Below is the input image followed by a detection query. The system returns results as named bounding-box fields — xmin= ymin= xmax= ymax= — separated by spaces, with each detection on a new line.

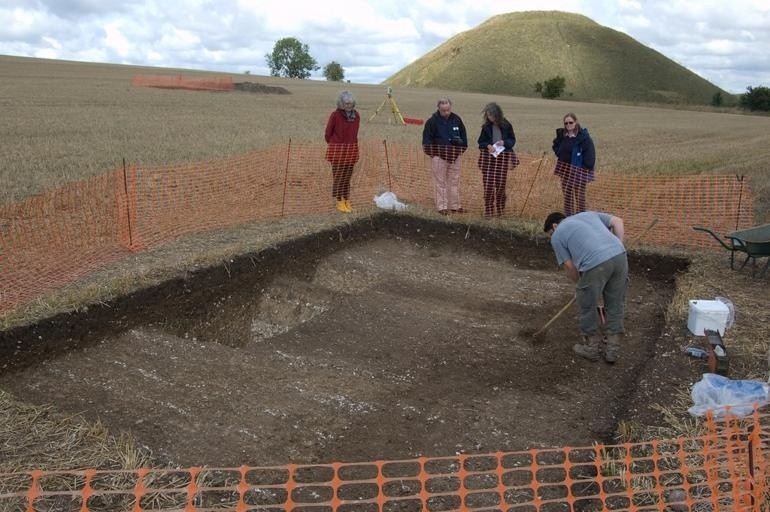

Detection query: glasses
xmin=564 ymin=122 xmax=574 ymax=125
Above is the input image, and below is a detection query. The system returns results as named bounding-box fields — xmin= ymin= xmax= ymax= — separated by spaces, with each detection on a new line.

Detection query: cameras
xmin=449 ymin=137 xmax=466 ymax=148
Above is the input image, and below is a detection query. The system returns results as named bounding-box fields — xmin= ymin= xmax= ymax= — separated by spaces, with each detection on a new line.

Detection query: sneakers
xmin=336 ymin=200 xmax=353 ymax=214
xmin=451 ymin=209 xmax=467 ymax=214
xmin=439 ymin=209 xmax=448 ymax=215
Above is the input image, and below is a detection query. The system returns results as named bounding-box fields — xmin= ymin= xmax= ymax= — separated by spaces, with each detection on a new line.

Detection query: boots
xmin=601 ymin=334 xmax=625 ymax=362
xmin=574 ymin=335 xmax=599 ymax=362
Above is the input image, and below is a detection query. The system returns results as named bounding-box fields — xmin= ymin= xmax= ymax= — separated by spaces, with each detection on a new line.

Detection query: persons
xmin=543 ymin=211 xmax=629 ymax=362
xmin=422 ymin=98 xmax=468 ymax=216
xmin=477 ymin=101 xmax=520 ymax=220
xmin=325 ymin=91 xmax=360 ymax=213
xmin=553 ymin=113 xmax=596 ymax=215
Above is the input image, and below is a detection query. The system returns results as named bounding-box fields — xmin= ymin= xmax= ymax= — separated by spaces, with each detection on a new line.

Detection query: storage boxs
xmin=687 ymin=299 xmax=729 ymax=337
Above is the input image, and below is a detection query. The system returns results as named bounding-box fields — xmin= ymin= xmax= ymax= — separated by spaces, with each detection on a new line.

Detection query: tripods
xmin=368 ymin=96 xmax=406 ymax=125
